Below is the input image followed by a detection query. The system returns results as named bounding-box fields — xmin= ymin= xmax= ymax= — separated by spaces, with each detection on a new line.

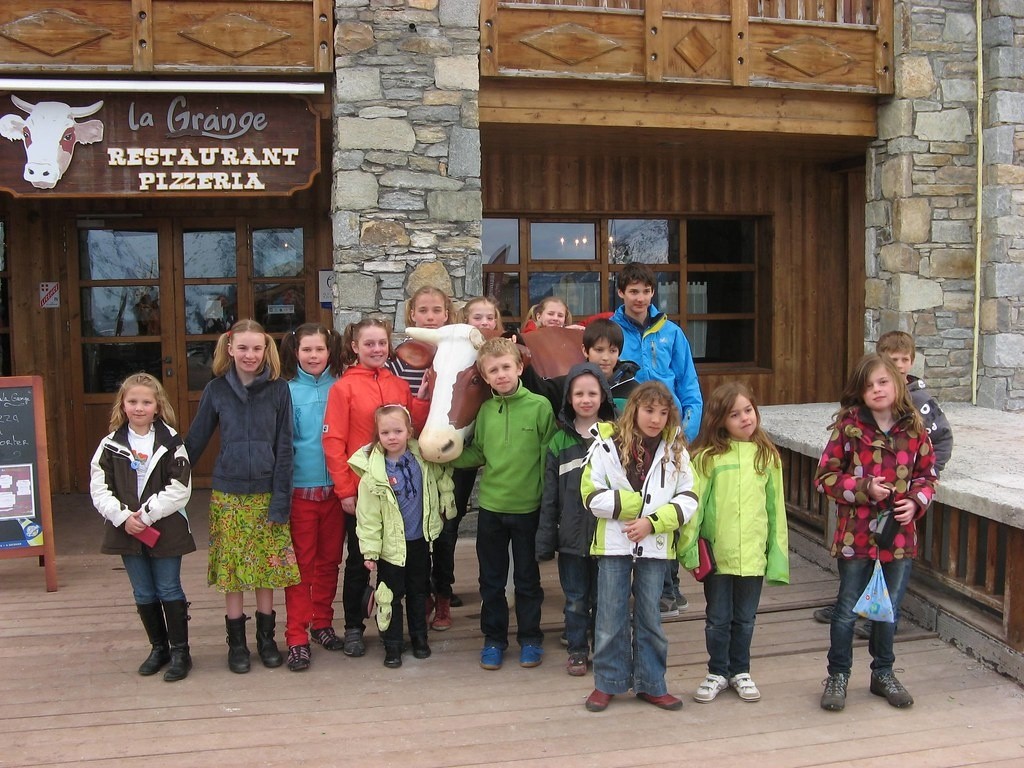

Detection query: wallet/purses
xmin=874 ymin=492 xmax=901 ymax=549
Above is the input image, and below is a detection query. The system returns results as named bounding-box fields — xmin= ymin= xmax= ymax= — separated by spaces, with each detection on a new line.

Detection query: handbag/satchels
xmin=853 ymin=559 xmax=894 ymax=624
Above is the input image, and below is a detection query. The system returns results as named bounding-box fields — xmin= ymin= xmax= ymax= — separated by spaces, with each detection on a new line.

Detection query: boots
xmin=137 ymin=607 xmax=170 ymax=675
xmin=226 ymin=613 xmax=250 ymax=673
xmin=255 ymin=610 xmax=282 ymax=667
xmin=162 ymin=600 xmax=192 ymax=681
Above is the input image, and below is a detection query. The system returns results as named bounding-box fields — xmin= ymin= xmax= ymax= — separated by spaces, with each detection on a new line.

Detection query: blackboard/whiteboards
xmin=0 ymin=376 xmax=53 ymax=559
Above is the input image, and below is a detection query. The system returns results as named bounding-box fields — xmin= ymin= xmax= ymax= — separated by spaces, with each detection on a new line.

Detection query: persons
xmin=813 ymin=353 xmax=936 ymax=713
xmin=181 ymin=262 xmax=702 ymax=676
xmin=814 ymin=332 xmax=953 ymax=639
xmin=356 ymin=404 xmax=455 ymax=668
xmin=676 ymin=382 xmax=788 ymax=704
xmin=89 ymin=373 xmax=192 ymax=681
xmin=580 ymin=383 xmax=700 ymax=712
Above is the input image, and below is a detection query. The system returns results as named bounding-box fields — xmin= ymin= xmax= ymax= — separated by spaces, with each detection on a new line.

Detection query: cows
xmin=394 ymin=321 xmax=586 ymax=462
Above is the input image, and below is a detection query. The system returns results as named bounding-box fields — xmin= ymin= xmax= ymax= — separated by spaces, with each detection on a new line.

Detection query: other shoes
xmin=814 ymin=606 xmax=835 ymax=622
xmin=855 ymin=621 xmax=872 ymax=638
xmin=449 ymin=592 xmax=463 ymax=606
xmin=676 ymin=593 xmax=690 ymax=609
xmin=659 ymin=596 xmax=679 ymax=618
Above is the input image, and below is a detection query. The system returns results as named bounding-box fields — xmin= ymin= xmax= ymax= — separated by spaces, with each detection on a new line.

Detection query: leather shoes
xmin=385 ymin=646 xmax=401 ymax=667
xmin=411 ymin=634 xmax=431 ymax=658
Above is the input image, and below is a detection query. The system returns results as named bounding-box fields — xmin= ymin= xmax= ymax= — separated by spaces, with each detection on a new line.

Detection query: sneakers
xmin=729 ymin=673 xmax=760 ymax=702
xmin=567 ymin=652 xmax=587 ymax=675
xmin=586 ymin=689 xmax=613 ymax=712
xmin=870 ymin=668 xmax=914 ymax=707
xmin=694 ymin=673 xmax=728 ymax=703
xmin=310 ymin=628 xmax=344 ymax=650
xmin=821 ymin=673 xmax=848 ymax=710
xmin=287 ymin=644 xmax=311 ymax=670
xmin=480 ymin=644 xmax=503 ymax=669
xmin=344 ymin=628 xmax=366 ymax=656
xmin=433 ymin=593 xmax=451 ymax=630
xmin=520 ymin=642 xmax=543 ymax=666
xmin=425 ymin=596 xmax=434 ymax=630
xmin=637 ymin=693 xmax=683 ymax=710
